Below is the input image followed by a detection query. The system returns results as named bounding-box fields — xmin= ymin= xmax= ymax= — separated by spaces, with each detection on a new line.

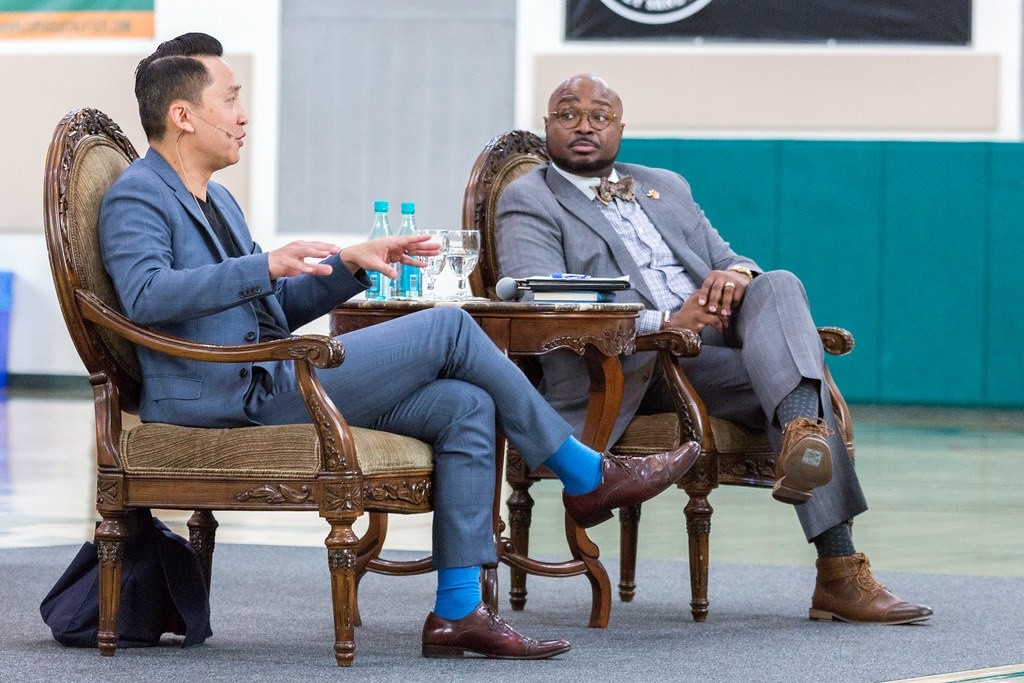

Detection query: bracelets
xmin=662 ymin=310 xmax=671 ymax=328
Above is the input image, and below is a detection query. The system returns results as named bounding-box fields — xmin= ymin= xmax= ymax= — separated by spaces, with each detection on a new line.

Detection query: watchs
xmin=727 ymin=266 xmax=753 ymax=281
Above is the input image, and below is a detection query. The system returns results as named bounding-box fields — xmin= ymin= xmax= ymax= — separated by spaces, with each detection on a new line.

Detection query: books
xmin=516 ymin=274 xmax=634 ymax=303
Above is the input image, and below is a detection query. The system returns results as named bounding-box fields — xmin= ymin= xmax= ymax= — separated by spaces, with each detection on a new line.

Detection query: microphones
xmin=495 ymin=277 xmax=525 ymax=302
xmin=187 ymin=106 xmax=234 ymax=137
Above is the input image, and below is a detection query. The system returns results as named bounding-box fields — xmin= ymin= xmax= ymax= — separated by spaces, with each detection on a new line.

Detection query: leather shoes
xmin=808 ymin=552 xmax=935 ymax=625
xmin=562 ymin=441 xmax=702 ymax=529
xmin=771 ymin=417 xmax=833 ymax=507
xmin=421 ymin=600 xmax=572 ymax=659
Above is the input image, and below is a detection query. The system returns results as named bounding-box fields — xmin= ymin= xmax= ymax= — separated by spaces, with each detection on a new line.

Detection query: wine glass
xmin=416 ymin=230 xmax=450 ymax=299
xmin=449 ymin=230 xmax=480 ymax=297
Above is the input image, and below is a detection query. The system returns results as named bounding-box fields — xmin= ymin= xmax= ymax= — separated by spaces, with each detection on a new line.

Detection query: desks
xmin=330 ymin=296 xmax=644 ymax=627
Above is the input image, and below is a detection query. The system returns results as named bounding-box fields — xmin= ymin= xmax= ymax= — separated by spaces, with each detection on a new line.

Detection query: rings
xmin=725 ymin=282 xmax=736 ymax=288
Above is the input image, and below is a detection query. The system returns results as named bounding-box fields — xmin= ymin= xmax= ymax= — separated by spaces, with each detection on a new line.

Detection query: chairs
xmin=463 ymin=129 xmax=854 ymax=622
xmin=43 ymin=106 xmax=435 ymax=666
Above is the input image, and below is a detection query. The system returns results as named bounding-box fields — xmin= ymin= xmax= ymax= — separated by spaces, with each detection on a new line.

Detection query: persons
xmin=97 ymin=33 xmax=700 ymax=659
xmin=495 ymin=76 xmax=935 ymax=628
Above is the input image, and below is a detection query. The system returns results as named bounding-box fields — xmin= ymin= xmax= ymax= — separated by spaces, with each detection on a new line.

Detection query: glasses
xmin=548 ymin=107 xmax=621 ymax=131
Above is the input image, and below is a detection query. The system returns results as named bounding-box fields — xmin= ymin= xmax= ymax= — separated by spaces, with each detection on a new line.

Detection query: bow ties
xmin=589 ymin=173 xmax=635 ymax=205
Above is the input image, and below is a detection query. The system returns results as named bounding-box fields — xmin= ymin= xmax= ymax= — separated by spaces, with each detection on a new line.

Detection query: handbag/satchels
xmin=43 ymin=509 xmax=214 ymax=651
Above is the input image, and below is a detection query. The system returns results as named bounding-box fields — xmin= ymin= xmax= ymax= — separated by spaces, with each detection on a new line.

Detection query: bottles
xmin=396 ymin=202 xmax=422 ymax=298
xmin=365 ymin=200 xmax=396 ymax=300
